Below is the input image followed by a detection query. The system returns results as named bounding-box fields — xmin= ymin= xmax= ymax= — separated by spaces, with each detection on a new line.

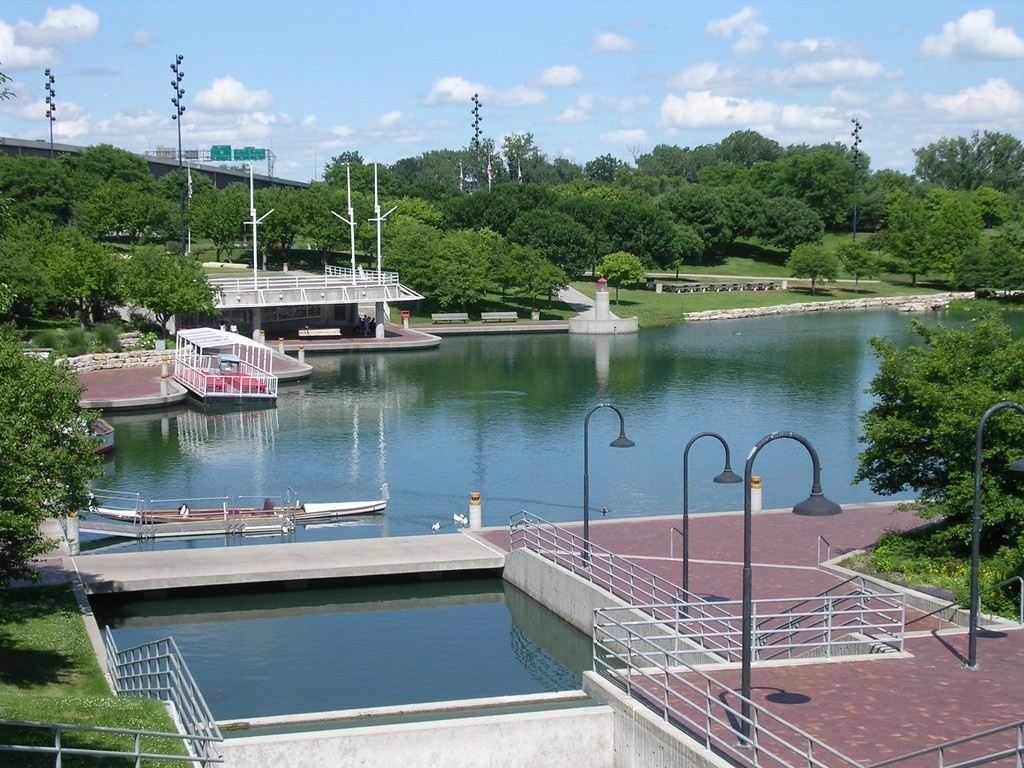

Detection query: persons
xmin=354 ymin=315 xmax=375 ymax=336
xmin=230 ymin=321 xmax=238 ymax=333
xmin=219 ymin=320 xmax=228 ymax=331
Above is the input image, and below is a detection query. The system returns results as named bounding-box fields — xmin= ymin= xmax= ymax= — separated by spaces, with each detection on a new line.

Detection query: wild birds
xmin=733 ymin=331 xmax=742 ymax=336
xmin=432 ymin=522 xmax=440 ymax=531
xmin=454 ymin=513 xmax=468 ymax=524
xmin=602 ymin=507 xmax=608 ymax=513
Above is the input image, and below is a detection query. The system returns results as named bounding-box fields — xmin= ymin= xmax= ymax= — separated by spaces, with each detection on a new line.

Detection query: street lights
xmin=171 ymin=53 xmax=188 ymax=254
xmin=680 ymin=431 xmax=744 ymax=623
xmin=851 ymin=115 xmax=863 ymax=240
xmin=45 ymin=68 xmax=56 ymax=156
xmin=471 ymin=92 xmax=483 ymax=187
xmin=738 ymin=429 xmax=844 ymax=749
xmin=582 ymin=402 xmax=636 ymax=571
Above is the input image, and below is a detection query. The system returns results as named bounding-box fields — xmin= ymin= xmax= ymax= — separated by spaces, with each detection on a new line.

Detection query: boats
xmin=89 ymin=498 xmax=388 ymax=526
xmin=61 ymin=413 xmax=115 ymax=461
